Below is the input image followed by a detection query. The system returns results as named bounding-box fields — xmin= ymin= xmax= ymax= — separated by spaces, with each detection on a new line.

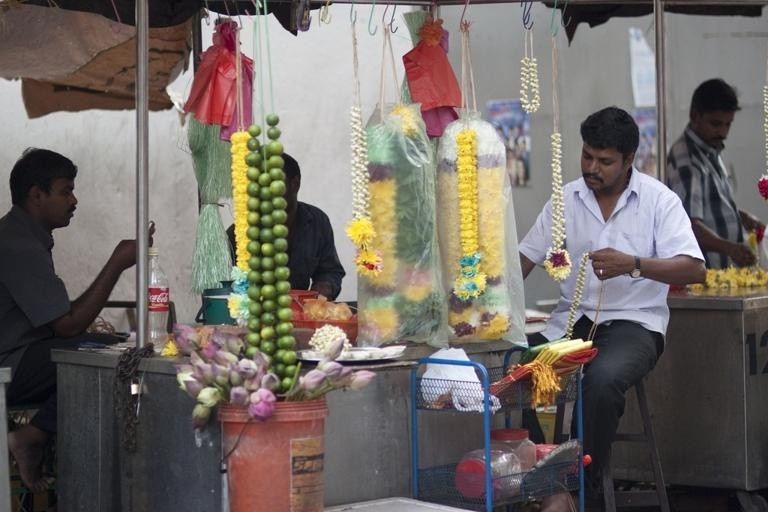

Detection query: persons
xmin=224 ymin=148 xmax=348 ymax=302
xmin=662 ymin=74 xmax=768 ymax=270
xmin=510 ymin=105 xmax=708 ymax=473
xmin=2 ymin=140 xmax=156 ymax=490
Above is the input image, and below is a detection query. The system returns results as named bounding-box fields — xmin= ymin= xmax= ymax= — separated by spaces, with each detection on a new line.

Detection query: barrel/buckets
xmin=218 ymin=400 xmax=330 ymax=511
xmin=285 ymin=290 xmax=319 ymax=318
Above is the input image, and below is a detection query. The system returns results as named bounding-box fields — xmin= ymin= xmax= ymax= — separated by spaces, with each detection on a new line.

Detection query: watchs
xmin=629 ymin=254 xmax=644 ymax=277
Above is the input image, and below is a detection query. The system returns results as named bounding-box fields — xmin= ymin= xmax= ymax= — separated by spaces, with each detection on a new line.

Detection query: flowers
xmin=170 ymin=321 xmax=377 ymax=431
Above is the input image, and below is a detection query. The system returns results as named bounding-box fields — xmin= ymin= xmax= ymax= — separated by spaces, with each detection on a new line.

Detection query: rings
xmin=599 ymin=269 xmax=603 ymax=277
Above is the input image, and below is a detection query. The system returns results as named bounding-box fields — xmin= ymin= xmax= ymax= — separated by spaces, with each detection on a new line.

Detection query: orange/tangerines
xmin=244 ymin=112 xmax=297 ymax=392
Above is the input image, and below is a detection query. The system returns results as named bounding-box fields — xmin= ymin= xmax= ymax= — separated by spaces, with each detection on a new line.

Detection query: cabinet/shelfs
xmin=412 ymin=345 xmax=585 ymax=512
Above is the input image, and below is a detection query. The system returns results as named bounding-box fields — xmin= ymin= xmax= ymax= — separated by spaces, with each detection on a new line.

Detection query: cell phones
xmin=148 ymin=221 xmax=155 ymax=239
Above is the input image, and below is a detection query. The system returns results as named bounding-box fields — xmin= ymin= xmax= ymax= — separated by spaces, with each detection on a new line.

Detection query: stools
xmin=554 ymin=363 xmax=669 ymax=511
xmin=9 ymin=404 xmax=55 ymax=511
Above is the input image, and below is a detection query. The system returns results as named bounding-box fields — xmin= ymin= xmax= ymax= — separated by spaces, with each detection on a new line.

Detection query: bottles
xmin=455 ymin=446 xmax=522 ymax=501
xmin=147 ymin=245 xmax=172 ymax=349
xmin=488 ymin=426 xmax=537 ymax=473
